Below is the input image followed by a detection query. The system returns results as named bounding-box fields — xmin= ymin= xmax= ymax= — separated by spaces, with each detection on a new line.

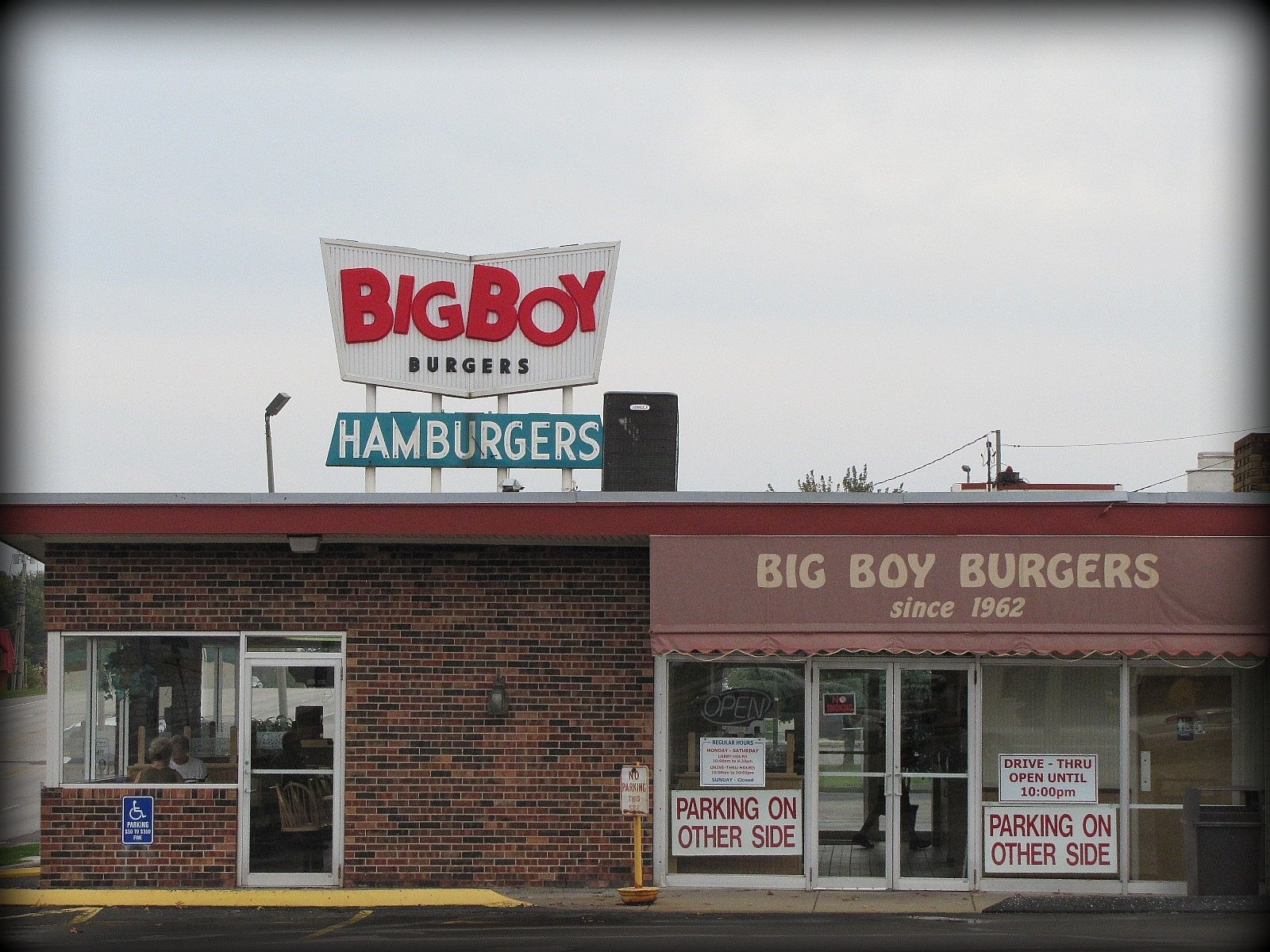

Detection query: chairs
xmin=278 ymin=783 xmax=326 ymax=872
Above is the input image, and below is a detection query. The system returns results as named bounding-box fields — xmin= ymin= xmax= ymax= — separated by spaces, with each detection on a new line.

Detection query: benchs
xmin=126 ymin=764 xmax=239 ymax=786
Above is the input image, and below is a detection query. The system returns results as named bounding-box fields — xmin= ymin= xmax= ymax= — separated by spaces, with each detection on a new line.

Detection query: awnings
xmin=648 ymin=531 xmax=1270 ymax=672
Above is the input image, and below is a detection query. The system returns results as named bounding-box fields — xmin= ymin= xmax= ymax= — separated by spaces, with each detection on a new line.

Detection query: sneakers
xmin=852 ymin=833 xmax=875 ymax=849
xmin=909 ymin=838 xmax=932 ymax=849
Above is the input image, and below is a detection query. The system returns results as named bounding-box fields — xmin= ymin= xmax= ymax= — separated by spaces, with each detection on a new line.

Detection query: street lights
xmin=265 ymin=390 xmax=289 ymax=492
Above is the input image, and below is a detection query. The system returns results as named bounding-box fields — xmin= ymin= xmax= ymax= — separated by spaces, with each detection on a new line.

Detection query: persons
xmin=269 ymin=730 xmax=310 ymax=809
xmin=132 ymin=736 xmax=187 ymax=785
xmin=850 ymin=700 xmax=934 ymax=851
xmin=164 ymin=735 xmax=212 ymax=784
